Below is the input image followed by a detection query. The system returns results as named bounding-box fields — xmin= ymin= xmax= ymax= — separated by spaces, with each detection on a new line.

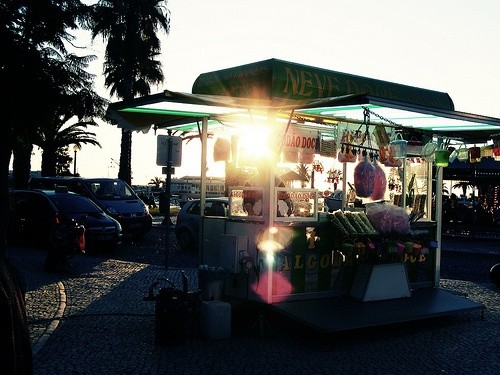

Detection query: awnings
xmin=274 ymin=91 xmax=500 ymax=134
xmin=104 ymin=89 xmax=270 ymax=134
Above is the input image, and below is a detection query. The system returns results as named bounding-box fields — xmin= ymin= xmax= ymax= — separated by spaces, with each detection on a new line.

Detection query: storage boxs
xmin=200 ymin=300 xmax=233 ymax=340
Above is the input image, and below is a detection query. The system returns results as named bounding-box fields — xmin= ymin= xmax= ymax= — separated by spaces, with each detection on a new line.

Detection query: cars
xmin=177 ymin=196 xmax=244 ymax=251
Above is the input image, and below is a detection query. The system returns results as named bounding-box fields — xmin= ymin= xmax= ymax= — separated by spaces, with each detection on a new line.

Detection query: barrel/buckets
xmin=202 ymin=299 xmax=231 ymax=339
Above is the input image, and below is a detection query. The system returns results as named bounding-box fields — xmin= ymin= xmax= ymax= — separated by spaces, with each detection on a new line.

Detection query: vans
xmin=0 ymin=175 xmax=154 ymax=253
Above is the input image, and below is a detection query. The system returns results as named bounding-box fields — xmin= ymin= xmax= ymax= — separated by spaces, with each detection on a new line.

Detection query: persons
xmin=241 ymin=153 xmax=295 ymax=217
xmin=172 ymin=194 xmax=193 ymax=209
xmin=137 ymin=191 xmax=156 ymax=209
